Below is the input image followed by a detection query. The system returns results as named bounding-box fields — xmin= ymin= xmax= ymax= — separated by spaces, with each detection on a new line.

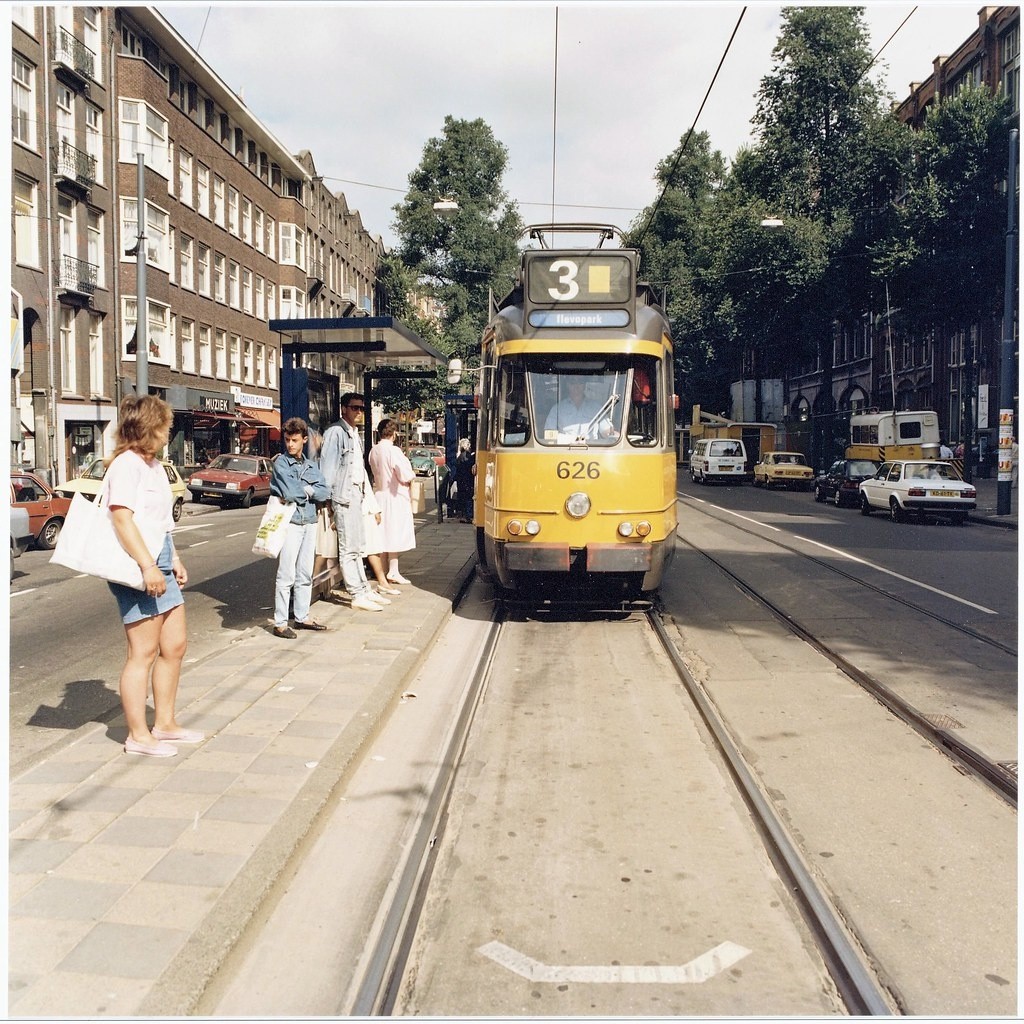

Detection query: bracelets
xmin=172 ymin=556 xmax=180 ymax=560
xmin=141 ymin=562 xmax=157 ymax=571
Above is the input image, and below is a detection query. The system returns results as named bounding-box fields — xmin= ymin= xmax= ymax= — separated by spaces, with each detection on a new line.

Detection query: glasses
xmin=346 ymin=405 xmax=367 ymax=412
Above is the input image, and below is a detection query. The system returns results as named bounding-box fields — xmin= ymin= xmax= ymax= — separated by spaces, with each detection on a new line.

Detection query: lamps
xmin=759 ymin=215 xmax=785 ymax=231
xmin=432 ymin=195 xmax=459 ymax=215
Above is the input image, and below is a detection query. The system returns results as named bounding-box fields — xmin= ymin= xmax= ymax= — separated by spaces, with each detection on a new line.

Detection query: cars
xmin=53 ymin=459 xmax=186 ymax=522
xmin=813 ymin=459 xmax=900 ymax=508
xmin=10 ymin=472 xmax=72 ymax=549
xmin=753 ymin=452 xmax=814 ymax=492
xmin=187 ymin=453 xmax=273 ymax=508
xmin=858 ymin=459 xmax=978 ymax=526
xmin=400 ymin=441 xmax=446 ymax=477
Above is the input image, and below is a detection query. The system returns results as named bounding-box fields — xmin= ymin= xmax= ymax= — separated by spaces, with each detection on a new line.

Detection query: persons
xmin=940 ymin=437 xmax=964 ymax=458
xmin=269 ymin=417 xmax=328 ymax=639
xmin=544 ymin=376 xmax=619 ymax=440
xmin=99 ymin=390 xmax=204 ymax=757
xmin=319 ymin=392 xmax=392 ymax=611
xmin=456 ymin=438 xmax=475 ymax=523
xmin=368 ymin=418 xmax=416 ymax=584
xmin=1012 ymin=437 xmax=1018 ymax=489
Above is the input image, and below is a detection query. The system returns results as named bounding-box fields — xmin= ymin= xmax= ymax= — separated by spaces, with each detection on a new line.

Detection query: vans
xmin=688 ymin=438 xmax=748 ymax=486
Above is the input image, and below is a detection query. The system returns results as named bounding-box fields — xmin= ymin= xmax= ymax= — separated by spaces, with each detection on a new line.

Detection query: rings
xmin=153 ymin=587 xmax=157 ymax=590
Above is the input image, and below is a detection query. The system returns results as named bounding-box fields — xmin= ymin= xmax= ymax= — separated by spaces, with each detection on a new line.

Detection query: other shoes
xmin=365 ymin=589 xmax=391 ymax=606
xmin=124 ymin=737 xmax=178 ymax=758
xmin=386 ymin=573 xmax=412 ymax=585
xmin=150 ymin=725 xmax=205 ymax=742
xmin=294 ymin=620 xmax=327 ymax=630
xmin=273 ymin=626 xmax=297 ymax=639
xmin=376 ymin=585 xmax=402 ymax=595
xmin=351 ymin=596 xmax=383 ymax=611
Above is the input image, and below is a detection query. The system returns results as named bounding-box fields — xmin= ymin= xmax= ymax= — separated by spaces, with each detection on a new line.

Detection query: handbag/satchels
xmin=251 ymin=495 xmax=297 ymax=560
xmin=48 ymin=468 xmax=167 ymax=592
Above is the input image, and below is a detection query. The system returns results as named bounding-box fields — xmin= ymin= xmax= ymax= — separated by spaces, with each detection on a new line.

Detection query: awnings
xmin=235 ymin=407 xmax=281 ymax=432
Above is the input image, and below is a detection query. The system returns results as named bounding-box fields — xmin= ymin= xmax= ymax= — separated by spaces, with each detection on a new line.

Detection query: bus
xmin=446 ymin=223 xmax=679 ymax=614
xmin=845 ymin=410 xmax=941 ymax=475
